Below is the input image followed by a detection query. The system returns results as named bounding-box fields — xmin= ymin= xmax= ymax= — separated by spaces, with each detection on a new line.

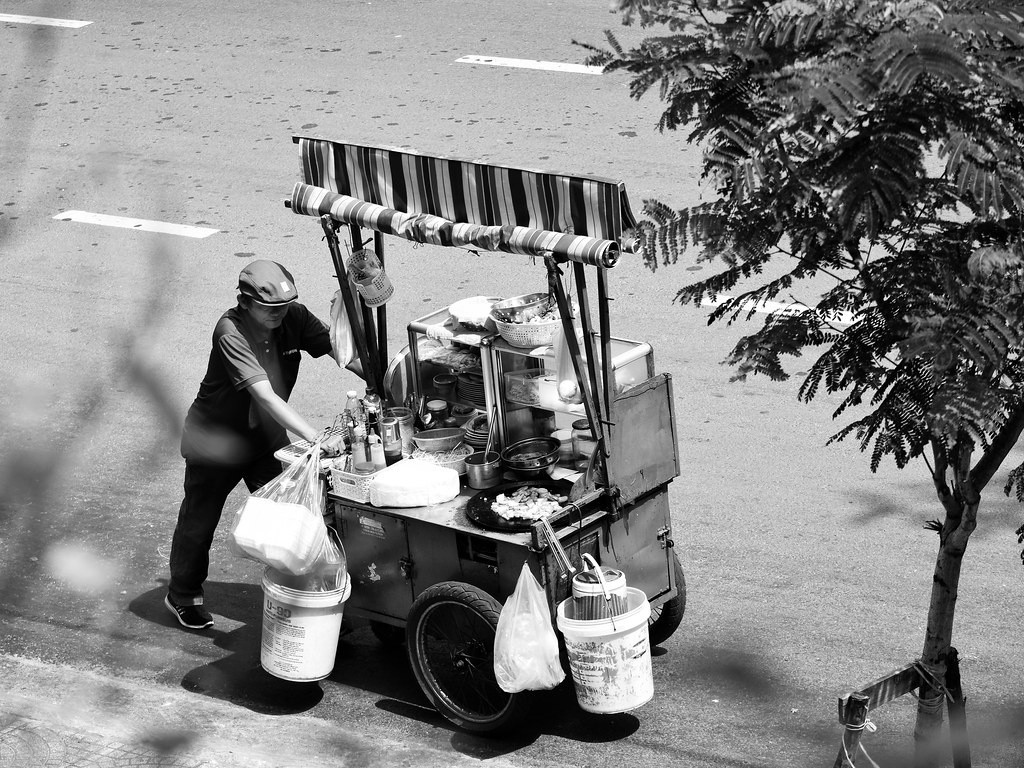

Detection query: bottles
xmin=346 ymin=388 xmax=386 ymax=472
xmin=571 ymin=421 xmax=597 ymax=473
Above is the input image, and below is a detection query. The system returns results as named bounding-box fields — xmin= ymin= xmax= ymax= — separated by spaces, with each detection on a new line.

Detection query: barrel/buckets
xmin=554 ymin=553 xmax=655 ymax=715
xmin=466 ymin=451 xmax=503 ymax=491
xmin=254 ymin=526 xmax=352 ymax=684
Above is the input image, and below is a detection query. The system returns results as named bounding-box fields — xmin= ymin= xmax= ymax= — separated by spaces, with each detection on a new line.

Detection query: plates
xmin=459 ymin=415 xmax=492 ymax=450
xmin=457 ymin=373 xmax=486 ymax=407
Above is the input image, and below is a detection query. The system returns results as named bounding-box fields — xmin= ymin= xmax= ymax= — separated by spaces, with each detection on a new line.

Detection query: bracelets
xmin=310 ymin=431 xmax=324 ymax=446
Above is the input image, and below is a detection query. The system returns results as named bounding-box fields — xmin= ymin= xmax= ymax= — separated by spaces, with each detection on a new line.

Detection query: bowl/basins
xmin=434 ymin=374 xmax=456 ymax=393
xmin=410 ymin=427 xmax=473 ymax=476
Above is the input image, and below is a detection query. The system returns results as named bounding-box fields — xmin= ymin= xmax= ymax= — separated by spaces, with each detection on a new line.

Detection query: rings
xmin=335 ymin=449 xmax=340 ymax=456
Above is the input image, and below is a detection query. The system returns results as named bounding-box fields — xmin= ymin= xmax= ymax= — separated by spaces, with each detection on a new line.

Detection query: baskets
xmin=489 ymin=298 xmax=583 ymax=349
xmin=343 ymin=248 xmax=394 ymax=309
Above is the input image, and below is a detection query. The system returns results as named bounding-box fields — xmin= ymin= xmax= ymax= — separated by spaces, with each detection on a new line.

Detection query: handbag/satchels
xmin=233 ymin=436 xmax=330 ymax=575
xmin=490 ymin=559 xmax=563 ymax=691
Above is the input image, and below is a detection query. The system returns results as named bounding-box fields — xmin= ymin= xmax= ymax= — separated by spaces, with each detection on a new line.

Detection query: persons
xmin=163 ymin=259 xmax=367 ymax=631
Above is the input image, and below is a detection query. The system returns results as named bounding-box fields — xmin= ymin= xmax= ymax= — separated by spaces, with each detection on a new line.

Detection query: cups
xmin=381 ymin=408 xmax=412 ymax=462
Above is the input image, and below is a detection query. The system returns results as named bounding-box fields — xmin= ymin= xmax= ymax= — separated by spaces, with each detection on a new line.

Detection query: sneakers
xmin=165 ymin=592 xmax=214 ymax=628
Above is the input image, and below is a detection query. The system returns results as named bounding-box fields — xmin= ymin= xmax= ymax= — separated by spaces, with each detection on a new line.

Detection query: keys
xmin=401 ymin=567 xmax=408 ymax=577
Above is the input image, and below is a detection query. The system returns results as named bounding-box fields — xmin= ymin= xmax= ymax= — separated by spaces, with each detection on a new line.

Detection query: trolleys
xmin=273 ymin=132 xmax=687 ymax=740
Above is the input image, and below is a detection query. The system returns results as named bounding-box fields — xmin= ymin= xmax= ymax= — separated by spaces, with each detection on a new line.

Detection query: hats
xmin=239 ymin=261 xmax=298 ymax=307
